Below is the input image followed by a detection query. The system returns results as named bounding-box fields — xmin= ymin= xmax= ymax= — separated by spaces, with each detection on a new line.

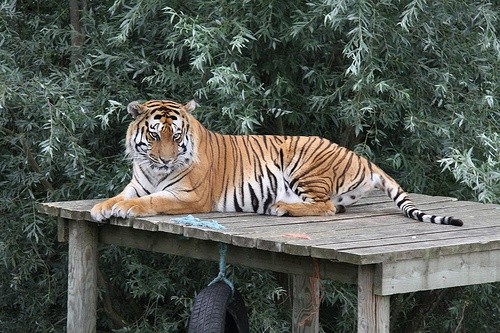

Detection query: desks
xmin=37 ymin=191 xmax=500 ymax=333
